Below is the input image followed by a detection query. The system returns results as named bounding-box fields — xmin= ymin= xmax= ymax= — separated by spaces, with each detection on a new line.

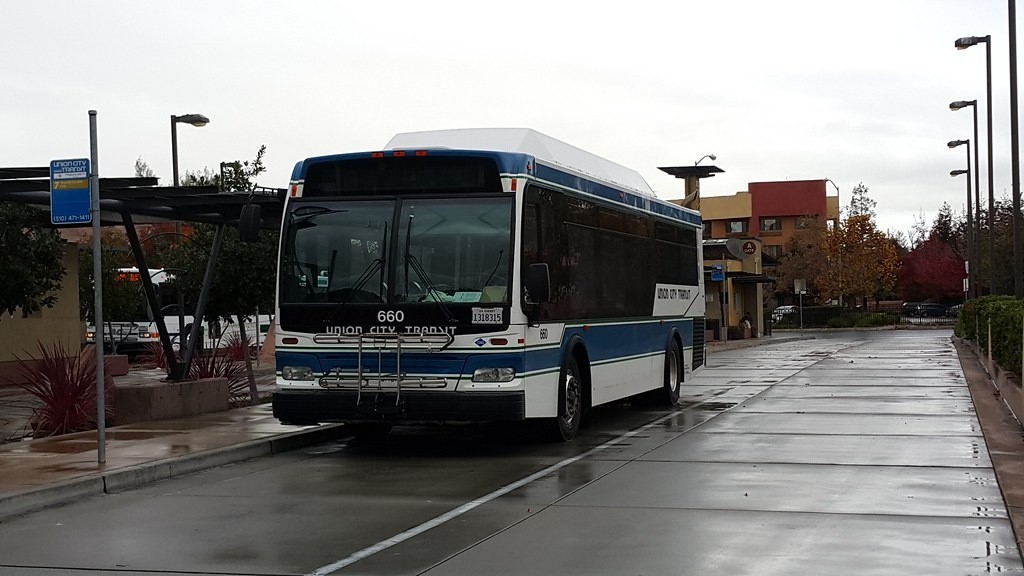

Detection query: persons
xmin=738 ymin=312 xmax=757 ymax=338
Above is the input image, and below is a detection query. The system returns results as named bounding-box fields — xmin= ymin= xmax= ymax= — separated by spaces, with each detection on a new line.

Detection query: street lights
xmin=824 ymin=178 xmax=844 ymax=306
xmin=955 ymin=31 xmax=998 ymax=295
xmin=944 ymin=98 xmax=982 ymax=300
xmin=169 ymin=111 xmax=209 ymax=359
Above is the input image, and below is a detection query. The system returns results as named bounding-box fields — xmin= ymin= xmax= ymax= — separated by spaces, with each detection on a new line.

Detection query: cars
xmin=771 ymin=305 xmax=800 ymax=323
xmin=945 ymin=304 xmax=964 ymax=317
xmin=902 ymin=296 xmax=948 ymax=317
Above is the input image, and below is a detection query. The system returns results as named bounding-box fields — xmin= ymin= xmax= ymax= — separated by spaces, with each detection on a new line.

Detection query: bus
xmin=265 ymin=123 xmax=710 ymax=445
xmin=83 ymin=266 xmax=183 ymax=360
